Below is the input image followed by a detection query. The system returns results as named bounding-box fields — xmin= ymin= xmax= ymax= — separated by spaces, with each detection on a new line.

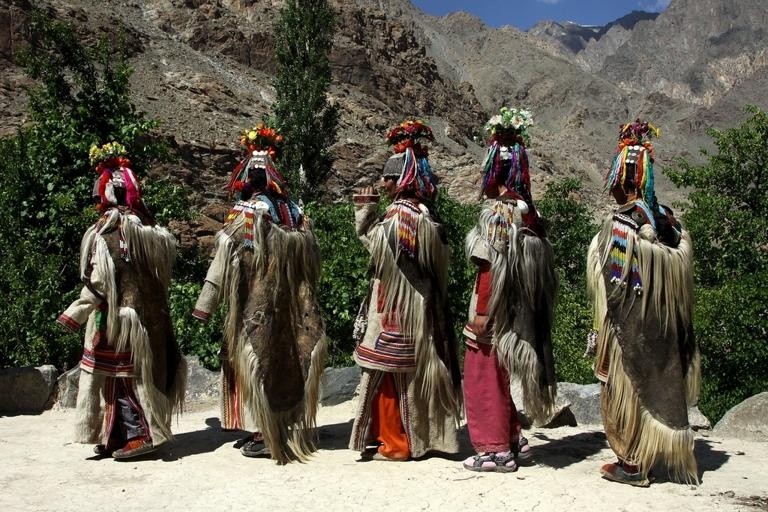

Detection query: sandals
xmin=463 ymin=437 xmax=531 ymax=473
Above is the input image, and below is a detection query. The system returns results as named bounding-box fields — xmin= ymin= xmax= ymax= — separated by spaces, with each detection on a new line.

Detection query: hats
xmin=244 ymin=150 xmax=275 ymax=170
xmin=625 ymin=145 xmax=646 ymax=165
xmin=382 ymin=152 xmax=406 ymax=177
xmin=92 ymin=168 xmax=140 ymax=198
xmin=499 ymin=144 xmax=514 ymax=160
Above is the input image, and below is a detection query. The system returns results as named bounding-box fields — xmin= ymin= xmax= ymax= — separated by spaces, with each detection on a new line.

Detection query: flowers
xmin=241 ymin=122 xmax=286 ymax=157
xmin=618 ymin=119 xmax=660 ymax=156
xmin=383 ymin=120 xmax=436 ymax=157
xmin=88 ymin=140 xmax=130 ymax=167
xmin=483 ymin=105 xmax=532 ymax=148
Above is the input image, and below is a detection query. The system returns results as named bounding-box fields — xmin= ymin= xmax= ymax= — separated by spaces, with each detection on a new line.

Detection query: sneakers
xmin=241 ymin=436 xmax=273 ymax=457
xmin=361 ymin=446 xmax=409 ymax=463
xmin=603 ymin=462 xmax=649 ymax=488
xmin=95 ymin=436 xmax=157 ymax=459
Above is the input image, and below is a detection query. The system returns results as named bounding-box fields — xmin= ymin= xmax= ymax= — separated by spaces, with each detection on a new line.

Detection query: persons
xmin=190 ymin=157 xmax=332 ymax=466
xmin=345 ymin=150 xmax=466 ymax=460
xmin=55 ymin=166 xmax=187 ymax=460
xmin=461 ymin=136 xmax=562 ymax=473
xmin=583 ymin=141 xmax=701 ymax=488
